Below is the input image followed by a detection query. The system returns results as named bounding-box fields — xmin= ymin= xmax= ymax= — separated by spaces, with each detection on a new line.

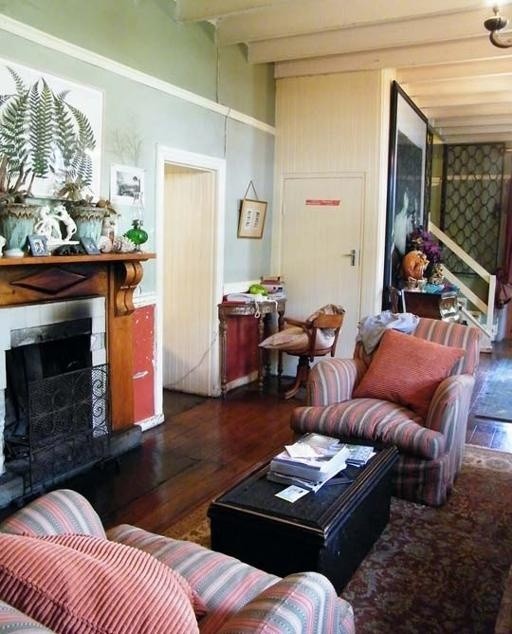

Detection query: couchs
xmin=290 ymin=317 xmax=480 ymax=507
xmin=0 ymin=489 xmax=356 ymax=634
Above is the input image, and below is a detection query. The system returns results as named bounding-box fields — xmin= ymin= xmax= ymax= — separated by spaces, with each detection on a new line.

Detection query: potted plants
xmin=0 ymin=66 xmax=54 ymax=250
xmin=97 ymin=200 xmax=121 ymax=243
xmin=51 ymin=90 xmax=106 ymax=242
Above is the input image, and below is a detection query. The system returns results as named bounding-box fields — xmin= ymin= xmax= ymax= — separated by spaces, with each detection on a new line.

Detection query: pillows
xmin=258 ymin=304 xmax=339 ymax=349
xmin=352 ymin=328 xmax=465 ymax=419
xmin=0 ymin=533 xmax=208 ymax=634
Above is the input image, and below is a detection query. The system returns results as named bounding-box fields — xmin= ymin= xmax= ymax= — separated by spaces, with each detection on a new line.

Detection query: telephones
xmin=227 ymin=293 xmax=260 ymax=318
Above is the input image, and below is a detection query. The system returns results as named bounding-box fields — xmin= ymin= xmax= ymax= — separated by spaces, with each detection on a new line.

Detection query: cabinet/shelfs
xmin=218 ymin=298 xmax=287 ymax=399
xmin=397 ymin=291 xmax=460 ymax=324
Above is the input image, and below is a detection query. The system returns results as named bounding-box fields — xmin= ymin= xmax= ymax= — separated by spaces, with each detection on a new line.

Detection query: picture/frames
xmin=80 ymin=238 xmax=101 ymax=254
xmin=237 ymin=198 xmax=268 ymax=239
xmin=27 ymin=234 xmax=49 ymax=256
xmin=109 ymin=163 xmax=145 ymax=208
xmin=382 ymin=80 xmax=428 ymax=311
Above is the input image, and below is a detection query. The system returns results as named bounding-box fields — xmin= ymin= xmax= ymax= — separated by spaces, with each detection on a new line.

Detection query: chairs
xmin=282 ymin=310 xmax=345 ymax=400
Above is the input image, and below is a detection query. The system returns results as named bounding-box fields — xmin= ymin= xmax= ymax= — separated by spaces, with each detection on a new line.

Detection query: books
xmin=268 ymin=433 xmax=349 ymax=493
xmin=344 ymin=444 xmax=377 ymax=467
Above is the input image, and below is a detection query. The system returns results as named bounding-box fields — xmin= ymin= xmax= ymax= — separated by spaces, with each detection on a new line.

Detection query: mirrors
xmin=0 ymin=59 xmax=104 ymax=201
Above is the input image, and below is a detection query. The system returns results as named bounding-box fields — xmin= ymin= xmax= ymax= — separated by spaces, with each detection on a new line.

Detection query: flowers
xmin=407 ymin=225 xmax=442 ymax=277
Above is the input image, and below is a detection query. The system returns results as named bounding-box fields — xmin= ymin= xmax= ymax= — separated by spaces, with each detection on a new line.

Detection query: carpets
xmin=158 ymin=442 xmax=511 ymax=634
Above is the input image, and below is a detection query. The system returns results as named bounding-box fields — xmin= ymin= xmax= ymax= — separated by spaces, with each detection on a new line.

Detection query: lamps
xmin=484 ymin=0 xmax=512 ymax=48
